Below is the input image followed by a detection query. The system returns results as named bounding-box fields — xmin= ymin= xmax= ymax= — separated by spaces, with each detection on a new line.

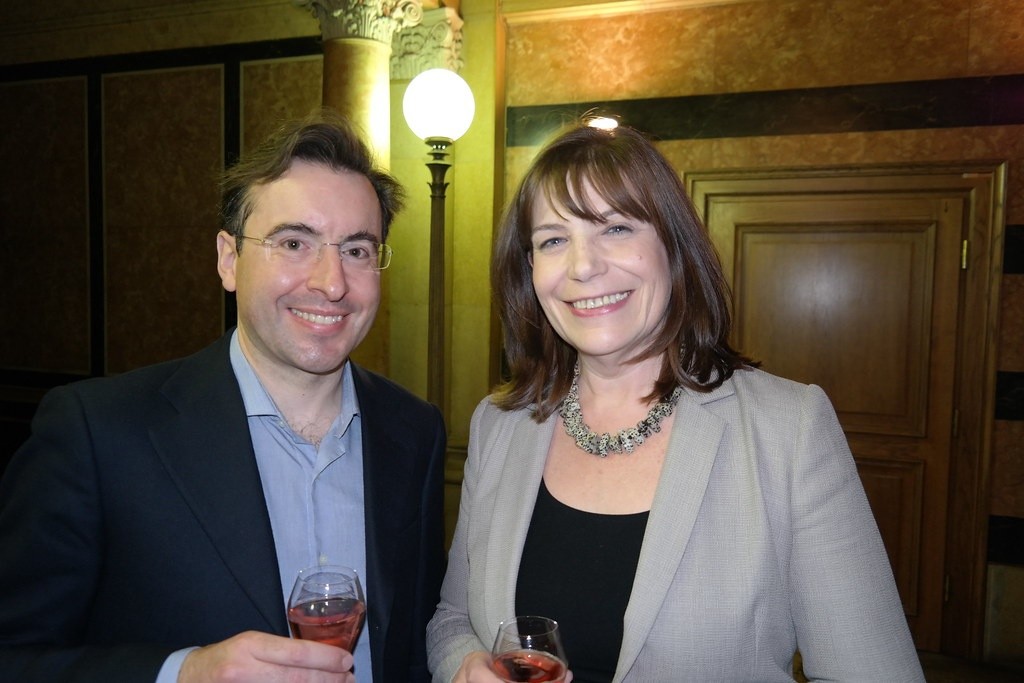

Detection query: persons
xmin=0 ymin=116 xmax=449 ymax=683
xmin=426 ymin=127 xmax=924 ymax=682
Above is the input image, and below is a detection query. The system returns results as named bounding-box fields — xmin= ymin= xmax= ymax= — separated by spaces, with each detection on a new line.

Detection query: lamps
xmin=402 ymin=68 xmax=476 ymax=420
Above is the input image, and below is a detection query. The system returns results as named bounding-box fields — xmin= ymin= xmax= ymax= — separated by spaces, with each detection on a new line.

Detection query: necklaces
xmin=559 ymin=361 xmax=683 ymax=457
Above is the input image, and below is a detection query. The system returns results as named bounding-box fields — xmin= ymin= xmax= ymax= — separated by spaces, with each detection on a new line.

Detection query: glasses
xmin=235 ymin=233 xmax=393 ymax=270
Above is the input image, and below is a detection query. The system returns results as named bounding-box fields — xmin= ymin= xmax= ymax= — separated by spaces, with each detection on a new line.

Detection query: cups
xmin=286 ymin=564 xmax=366 ymax=652
xmin=491 ymin=615 xmax=569 ymax=683
